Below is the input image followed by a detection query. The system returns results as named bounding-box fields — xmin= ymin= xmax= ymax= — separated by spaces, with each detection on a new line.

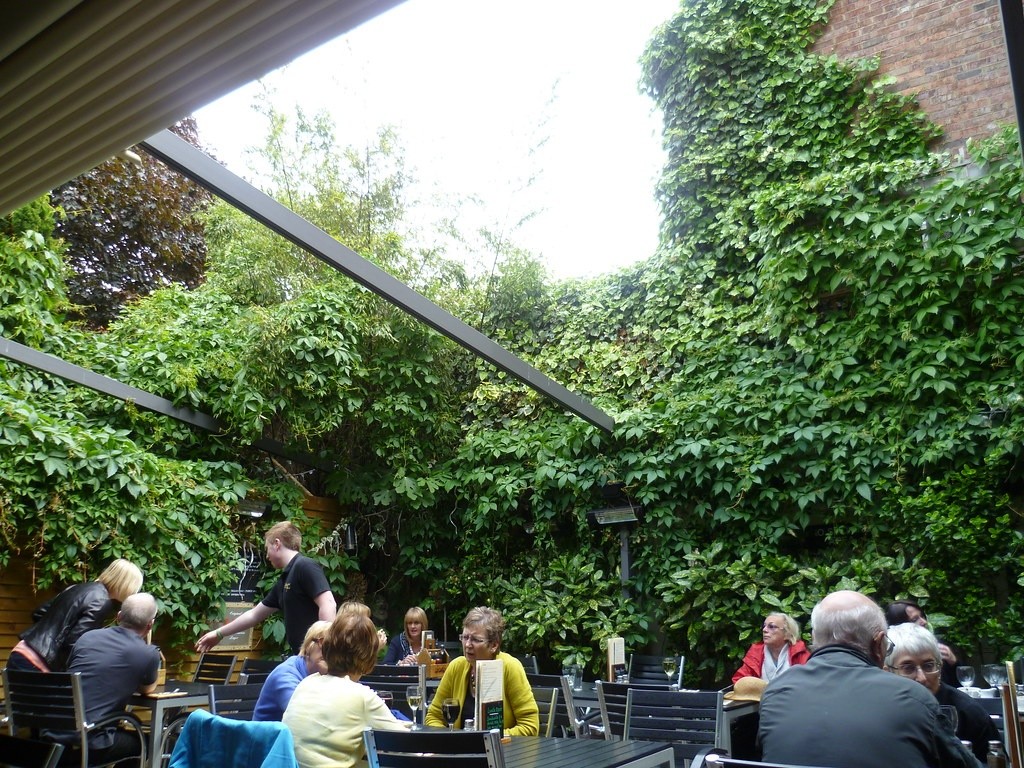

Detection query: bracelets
xmin=216 ymin=628 xmax=223 ymax=641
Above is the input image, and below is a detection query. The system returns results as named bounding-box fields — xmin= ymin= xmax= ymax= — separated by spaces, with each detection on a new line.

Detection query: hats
xmin=723 ymin=676 xmax=767 ymax=702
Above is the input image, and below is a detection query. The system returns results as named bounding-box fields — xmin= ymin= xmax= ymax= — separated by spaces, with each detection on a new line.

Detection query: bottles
xmin=986 ymin=740 xmax=1007 ymax=768
xmin=428 ymin=642 xmax=447 ymax=663
xmin=616 ymin=676 xmax=623 ymax=684
xmin=622 ymin=674 xmax=629 ymax=685
xmin=463 ymin=719 xmax=476 ymax=732
xmin=961 ymin=740 xmax=972 ymax=753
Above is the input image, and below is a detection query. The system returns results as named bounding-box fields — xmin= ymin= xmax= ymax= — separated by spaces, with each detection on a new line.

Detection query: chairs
xmin=0 ymin=641 xmax=1024 ymax=767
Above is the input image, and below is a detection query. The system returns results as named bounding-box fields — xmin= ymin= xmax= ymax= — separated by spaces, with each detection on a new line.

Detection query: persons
xmin=424 ymin=606 xmax=539 ymax=737
xmin=195 ymin=520 xmax=337 ymax=662
xmin=252 ymin=620 xmax=333 ymax=722
xmin=41 ymin=593 xmax=160 ymax=768
xmin=380 ymin=607 xmax=444 ymax=666
xmin=732 ymin=611 xmax=811 ymax=685
xmin=5 ymin=559 xmax=143 ymax=672
xmin=756 ymin=591 xmax=1011 ymax=768
xmin=281 ymin=601 xmax=413 ymax=768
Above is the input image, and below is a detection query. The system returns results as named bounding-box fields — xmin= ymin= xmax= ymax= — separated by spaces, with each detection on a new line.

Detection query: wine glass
xmin=956 ymin=666 xmax=975 ymax=697
xmin=662 ymin=657 xmax=676 ymax=686
xmin=406 ymin=685 xmax=424 ymax=730
xmin=443 ymin=699 xmax=460 ymax=731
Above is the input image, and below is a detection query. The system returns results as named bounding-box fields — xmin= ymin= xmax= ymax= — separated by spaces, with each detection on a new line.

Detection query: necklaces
xmin=413 ymin=641 xmax=419 ymax=646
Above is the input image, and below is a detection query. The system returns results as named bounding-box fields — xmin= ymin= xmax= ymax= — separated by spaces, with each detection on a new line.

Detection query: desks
xmin=568 ymin=682 xmax=761 ymax=757
xmin=115 ymin=684 xmax=220 ymax=767
xmin=502 ymin=732 xmax=676 ymax=766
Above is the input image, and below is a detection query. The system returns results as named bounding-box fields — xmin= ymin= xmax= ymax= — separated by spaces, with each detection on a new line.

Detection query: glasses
xmin=761 ymin=622 xmax=781 ymax=632
xmin=311 ymin=636 xmax=324 ymax=647
xmin=459 ymin=633 xmax=496 ymax=645
xmin=874 ymin=631 xmax=895 ymax=656
xmin=886 ymin=660 xmax=941 ymax=676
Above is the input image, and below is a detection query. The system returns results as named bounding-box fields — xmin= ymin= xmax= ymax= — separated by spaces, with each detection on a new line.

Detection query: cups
xmin=980 ymin=663 xmax=1008 ymax=688
xmin=562 ymin=664 xmax=583 ymax=690
xmin=377 ymin=691 xmax=393 ymax=713
